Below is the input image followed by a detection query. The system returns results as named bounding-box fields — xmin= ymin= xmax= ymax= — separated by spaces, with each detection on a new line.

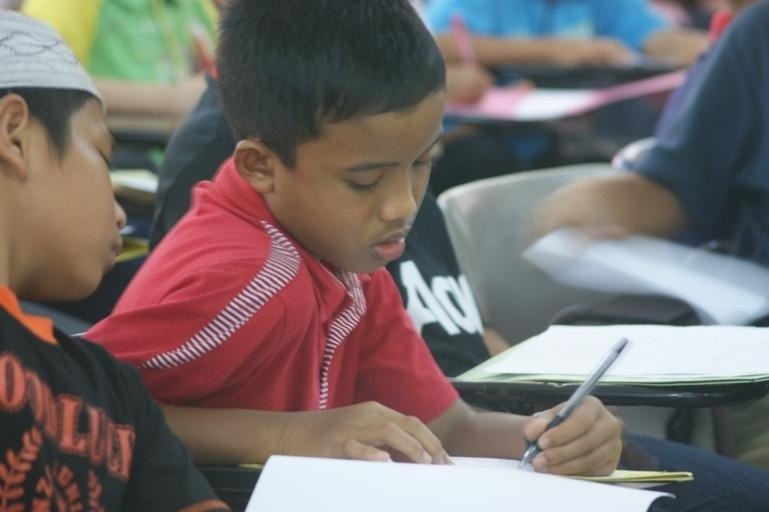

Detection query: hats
xmin=0 ymin=10 xmax=104 ymax=102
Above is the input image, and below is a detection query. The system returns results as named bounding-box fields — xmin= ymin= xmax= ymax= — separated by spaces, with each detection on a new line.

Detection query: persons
xmin=0 ymin=0 xmax=769 ymax=511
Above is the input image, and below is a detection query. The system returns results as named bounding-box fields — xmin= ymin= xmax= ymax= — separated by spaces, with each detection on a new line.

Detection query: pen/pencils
xmin=451 ymin=13 xmax=477 ymax=62
xmin=189 ymin=25 xmax=219 ymax=80
xmin=520 ymin=336 xmax=629 ymax=467
